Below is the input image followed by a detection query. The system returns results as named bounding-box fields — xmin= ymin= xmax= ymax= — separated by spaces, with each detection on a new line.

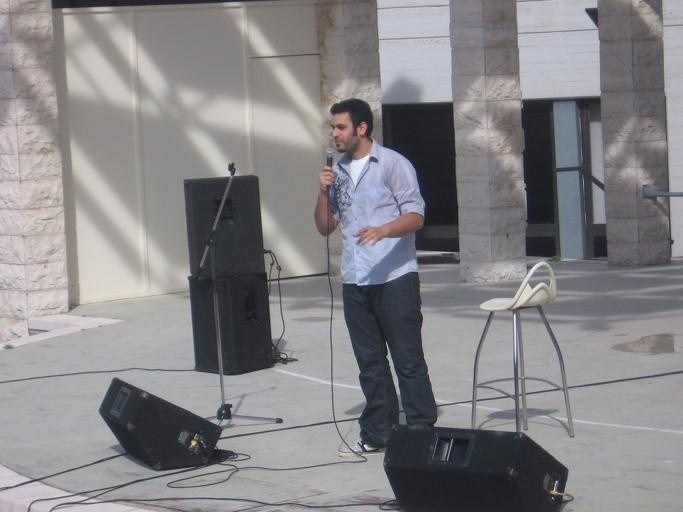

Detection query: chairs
xmin=470 ymin=261 xmax=574 ymax=437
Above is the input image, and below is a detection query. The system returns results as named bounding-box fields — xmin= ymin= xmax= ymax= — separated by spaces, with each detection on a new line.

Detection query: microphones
xmin=325 ymin=147 xmax=336 ymax=192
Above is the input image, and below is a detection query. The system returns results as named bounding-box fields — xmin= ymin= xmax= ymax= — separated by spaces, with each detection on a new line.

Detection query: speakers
xmin=187 ymin=272 xmax=274 ymax=376
xmin=382 ymin=423 xmax=569 ymax=511
xmin=98 ymin=377 xmax=223 ymax=471
xmin=184 ymin=174 xmax=265 ymax=278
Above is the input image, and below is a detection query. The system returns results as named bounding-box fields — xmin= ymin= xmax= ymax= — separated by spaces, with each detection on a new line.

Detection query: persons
xmin=311 ymin=97 xmax=439 ymax=458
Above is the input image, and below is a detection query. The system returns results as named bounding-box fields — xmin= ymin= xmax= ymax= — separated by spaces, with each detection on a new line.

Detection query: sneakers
xmin=336 ymin=437 xmax=385 ymax=457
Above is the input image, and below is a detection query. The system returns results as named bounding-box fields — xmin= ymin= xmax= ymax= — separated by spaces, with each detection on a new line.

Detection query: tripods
xmin=187 ymin=161 xmax=284 ymax=428
